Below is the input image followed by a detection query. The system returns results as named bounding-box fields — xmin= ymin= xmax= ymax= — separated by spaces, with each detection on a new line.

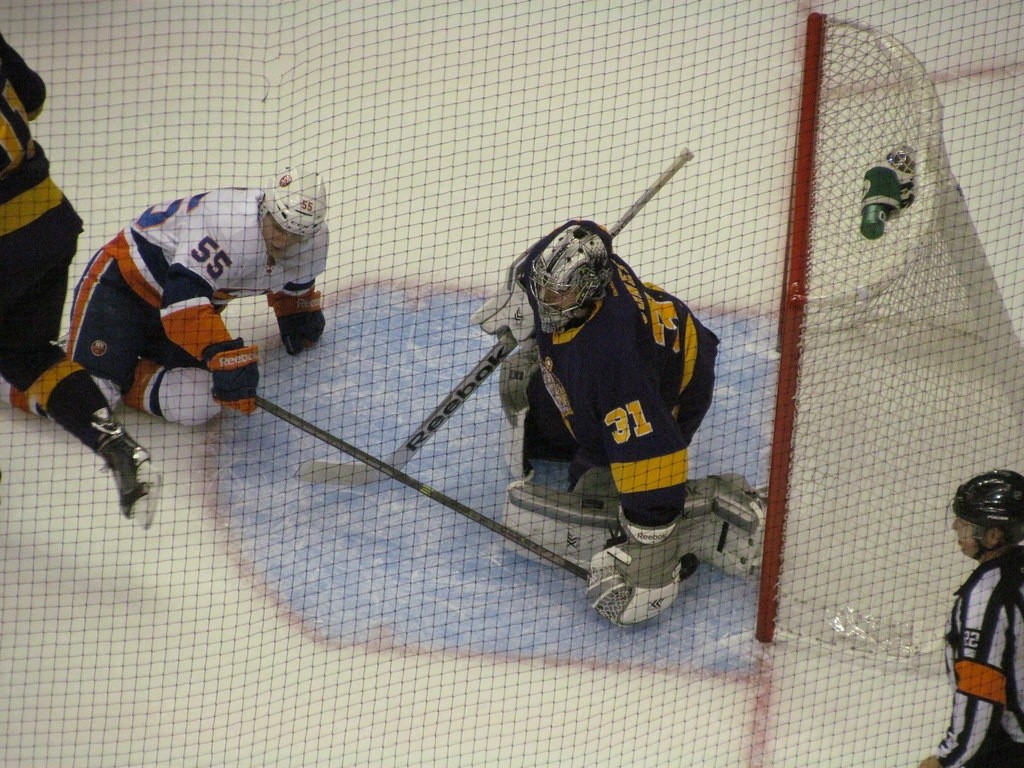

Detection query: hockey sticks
xmin=253 ymin=397 xmax=593 ymax=582
xmin=293 ymin=147 xmax=694 ymax=483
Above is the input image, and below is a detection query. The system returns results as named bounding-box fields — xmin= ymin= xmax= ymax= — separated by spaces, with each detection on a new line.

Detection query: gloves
xmin=272 ymin=290 xmax=326 ymax=356
xmin=587 ymin=505 xmax=683 ymax=628
xmin=471 ymin=276 xmax=540 ymax=348
xmin=204 ymin=344 xmax=260 ymax=416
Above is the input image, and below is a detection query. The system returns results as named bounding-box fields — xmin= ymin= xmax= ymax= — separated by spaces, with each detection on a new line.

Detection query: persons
xmin=917 ymin=469 xmax=1024 ymax=768
xmin=0 ymin=160 xmax=332 ymax=428
xmin=0 ymin=33 xmax=163 ymax=531
xmin=472 ymin=220 xmax=772 ymax=628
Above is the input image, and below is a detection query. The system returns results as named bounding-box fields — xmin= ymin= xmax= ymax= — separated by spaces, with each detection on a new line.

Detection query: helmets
xmin=257 ymin=164 xmax=328 ymax=238
xmin=531 ymin=218 xmax=614 ymax=332
xmin=953 ymin=468 xmax=1024 ymax=544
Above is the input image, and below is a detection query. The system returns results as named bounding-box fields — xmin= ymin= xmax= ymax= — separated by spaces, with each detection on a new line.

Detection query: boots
xmin=92 ymin=407 xmax=153 ymax=520
